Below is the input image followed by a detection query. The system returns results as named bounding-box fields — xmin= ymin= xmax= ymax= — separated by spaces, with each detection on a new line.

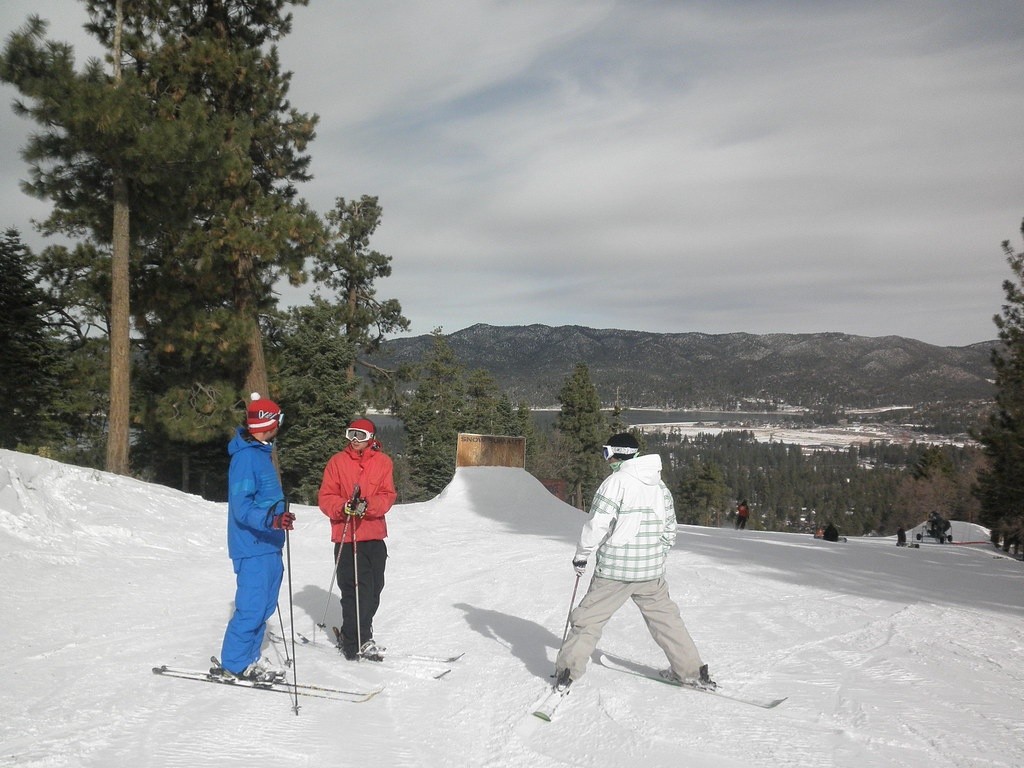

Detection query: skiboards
xmin=265 ymin=630 xmax=468 ymax=679
xmin=533 ymin=647 xmax=790 ymax=722
xmin=151 ymin=663 xmax=385 ymax=704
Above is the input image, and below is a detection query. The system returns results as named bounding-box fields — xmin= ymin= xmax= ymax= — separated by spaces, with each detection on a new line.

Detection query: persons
xmin=318 ymin=418 xmax=398 ymax=658
xmin=735 ymin=499 xmax=750 ymax=530
xmin=557 ymin=433 xmax=711 ymax=685
xmin=222 ymin=391 xmax=296 ymax=686
xmin=896 ymin=525 xmax=906 ymax=547
xmin=823 ymin=522 xmax=838 ymax=542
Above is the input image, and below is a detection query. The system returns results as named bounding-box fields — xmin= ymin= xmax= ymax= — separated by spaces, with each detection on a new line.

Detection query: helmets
xmin=350 ymin=419 xmax=376 ymax=446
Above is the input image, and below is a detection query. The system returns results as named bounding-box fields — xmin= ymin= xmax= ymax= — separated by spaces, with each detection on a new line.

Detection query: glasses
xmin=346 ymin=429 xmax=373 ymax=443
xmin=603 ymin=444 xmax=613 ymax=461
xmin=278 ymin=413 xmax=285 ymax=430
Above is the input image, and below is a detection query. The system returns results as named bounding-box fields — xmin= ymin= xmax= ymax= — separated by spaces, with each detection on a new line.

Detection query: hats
xmin=607 ymin=431 xmax=638 ymax=460
xmin=246 ymin=392 xmax=281 ymax=433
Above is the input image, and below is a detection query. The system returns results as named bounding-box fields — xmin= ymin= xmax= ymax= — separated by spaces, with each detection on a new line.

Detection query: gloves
xmin=273 ymin=513 xmax=296 ymax=530
xmin=344 ymin=500 xmax=367 ymax=520
xmin=573 ymin=555 xmax=587 ymax=577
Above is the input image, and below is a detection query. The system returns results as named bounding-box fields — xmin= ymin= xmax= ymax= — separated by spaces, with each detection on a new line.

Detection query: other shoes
xmin=219 ymin=659 xmax=285 ymax=687
xmin=660 ymin=664 xmax=710 ymax=687
xmin=557 ymin=670 xmax=574 ymax=692
xmin=342 ymin=639 xmax=384 ymax=661
